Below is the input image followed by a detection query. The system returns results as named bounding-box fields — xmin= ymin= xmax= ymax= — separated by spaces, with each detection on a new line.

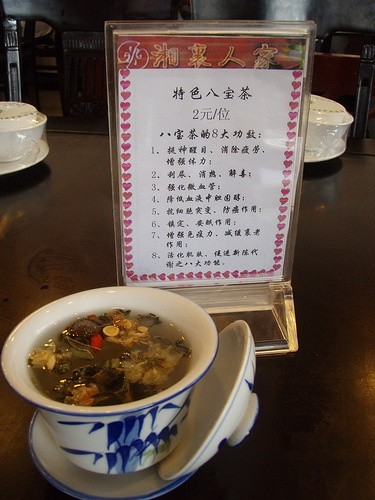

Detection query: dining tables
xmin=0 ymin=130 xmax=374 ymax=500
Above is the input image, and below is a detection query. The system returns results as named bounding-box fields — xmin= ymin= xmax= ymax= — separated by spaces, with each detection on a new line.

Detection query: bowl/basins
xmin=0 ymin=101 xmax=39 ymax=130
xmin=308 ymin=94 xmax=347 ymax=123
xmin=0 ymin=286 xmax=220 ymax=476
xmin=0 ymin=111 xmax=49 ymax=163
xmin=304 ymin=110 xmax=354 ymax=154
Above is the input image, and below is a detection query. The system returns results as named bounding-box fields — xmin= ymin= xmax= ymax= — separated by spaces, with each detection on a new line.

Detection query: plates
xmin=303 ymin=138 xmax=346 ymax=163
xmin=27 ymin=407 xmax=196 ymax=500
xmin=0 ymin=139 xmax=50 ymax=175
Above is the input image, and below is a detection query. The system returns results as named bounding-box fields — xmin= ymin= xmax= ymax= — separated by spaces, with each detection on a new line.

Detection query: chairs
xmin=313 ymin=45 xmax=375 ymax=138
